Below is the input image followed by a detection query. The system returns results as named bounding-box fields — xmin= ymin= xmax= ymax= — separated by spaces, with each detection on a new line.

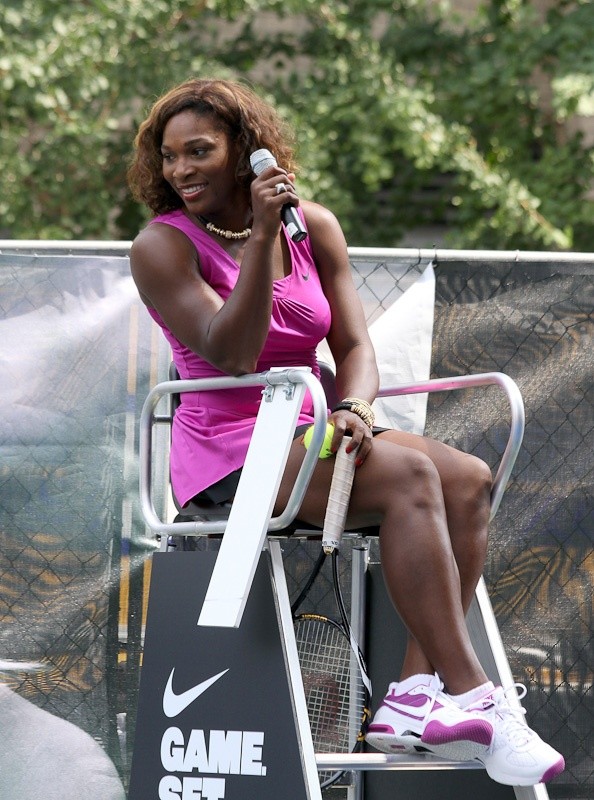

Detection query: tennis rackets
xmin=289 ymin=435 xmax=373 ymax=792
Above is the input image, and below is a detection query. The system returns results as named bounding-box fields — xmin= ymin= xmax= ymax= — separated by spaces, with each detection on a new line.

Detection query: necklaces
xmin=195 ymin=214 xmax=252 ymax=239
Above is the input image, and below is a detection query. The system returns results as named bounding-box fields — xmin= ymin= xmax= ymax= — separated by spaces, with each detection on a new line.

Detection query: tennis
xmin=303 ymin=424 xmax=334 ymax=458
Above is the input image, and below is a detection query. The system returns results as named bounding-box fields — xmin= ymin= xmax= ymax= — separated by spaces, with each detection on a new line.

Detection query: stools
xmin=137 ymin=356 xmax=549 ymax=800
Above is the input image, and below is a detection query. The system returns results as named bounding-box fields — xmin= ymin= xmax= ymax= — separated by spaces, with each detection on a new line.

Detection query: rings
xmin=274 ymin=182 xmax=287 ymax=195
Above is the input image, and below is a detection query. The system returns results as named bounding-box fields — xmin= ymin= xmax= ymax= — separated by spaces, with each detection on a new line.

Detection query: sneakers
xmin=365 ymin=673 xmax=493 ymax=762
xmin=460 ymin=683 xmax=565 ymax=787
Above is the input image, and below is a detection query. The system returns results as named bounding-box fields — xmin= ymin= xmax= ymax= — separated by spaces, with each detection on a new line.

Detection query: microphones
xmin=250 ymin=148 xmax=307 ymax=244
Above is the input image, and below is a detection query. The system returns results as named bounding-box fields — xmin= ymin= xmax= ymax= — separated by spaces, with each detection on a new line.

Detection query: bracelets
xmin=331 ymin=397 xmax=375 ymax=431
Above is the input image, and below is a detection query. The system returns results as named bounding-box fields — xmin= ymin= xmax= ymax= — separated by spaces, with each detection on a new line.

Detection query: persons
xmin=128 ymin=76 xmax=567 ymax=788
